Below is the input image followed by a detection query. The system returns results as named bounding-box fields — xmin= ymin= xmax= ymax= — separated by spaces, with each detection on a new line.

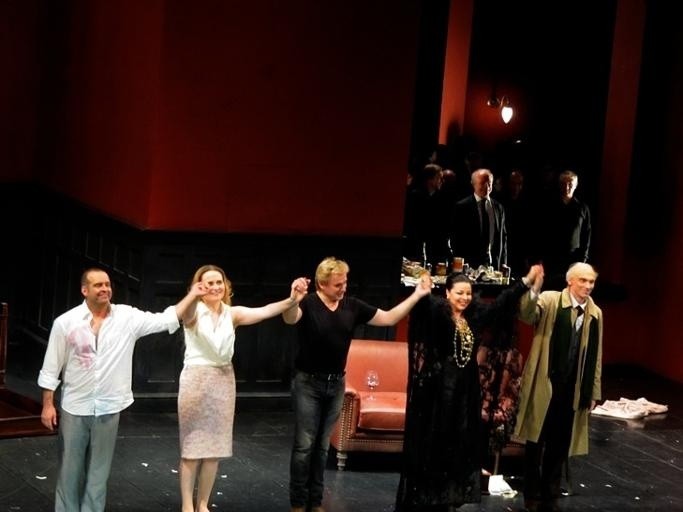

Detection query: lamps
xmin=486 ymin=95 xmax=514 ymax=127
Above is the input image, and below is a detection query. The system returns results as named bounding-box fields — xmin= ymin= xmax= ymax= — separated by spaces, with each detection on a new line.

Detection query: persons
xmin=33 ymin=267 xmax=210 ymax=511
xmin=509 ymin=261 xmax=604 ymax=511
xmin=472 ymin=340 xmax=503 ymax=406
xmin=478 ymin=347 xmax=525 ymax=423
xmin=393 ymin=264 xmax=540 ymax=511
xmin=283 ymin=254 xmax=434 ymax=511
xmin=164 ymin=264 xmax=310 ymax=511
xmin=403 ymin=149 xmax=596 ymax=290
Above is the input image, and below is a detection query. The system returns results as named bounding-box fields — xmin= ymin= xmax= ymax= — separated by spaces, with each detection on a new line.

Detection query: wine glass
xmin=363 ymin=368 xmax=380 ymax=403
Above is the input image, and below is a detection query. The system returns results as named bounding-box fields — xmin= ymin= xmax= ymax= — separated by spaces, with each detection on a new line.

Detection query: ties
xmin=480 ymin=199 xmax=490 ymax=247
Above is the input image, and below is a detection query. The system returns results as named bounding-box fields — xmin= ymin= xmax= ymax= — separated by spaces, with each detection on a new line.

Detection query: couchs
xmin=329 ymin=339 xmax=524 ymax=473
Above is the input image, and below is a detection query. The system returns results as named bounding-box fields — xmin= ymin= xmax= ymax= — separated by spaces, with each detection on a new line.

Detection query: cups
xmin=453 ymin=257 xmax=469 ymax=273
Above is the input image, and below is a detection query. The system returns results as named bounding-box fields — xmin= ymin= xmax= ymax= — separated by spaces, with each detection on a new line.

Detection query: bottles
xmin=435 ymin=262 xmax=447 ymax=276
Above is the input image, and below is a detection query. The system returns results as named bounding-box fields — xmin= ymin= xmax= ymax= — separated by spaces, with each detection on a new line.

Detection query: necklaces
xmin=453 ymin=317 xmax=475 ymax=368
